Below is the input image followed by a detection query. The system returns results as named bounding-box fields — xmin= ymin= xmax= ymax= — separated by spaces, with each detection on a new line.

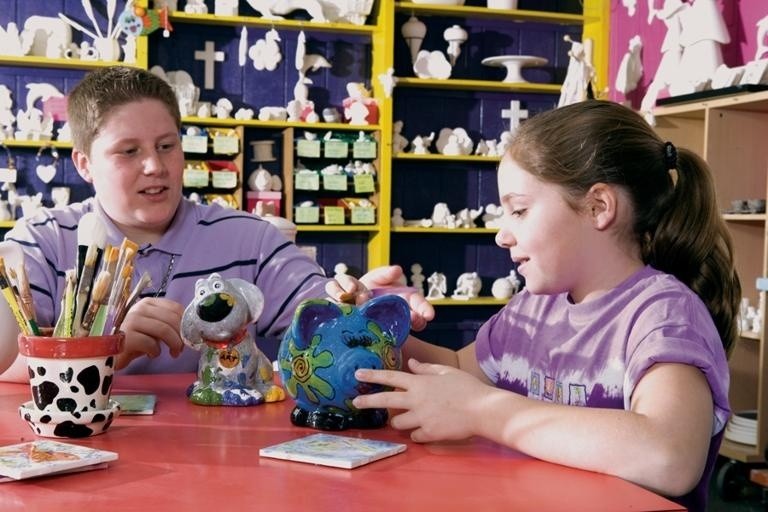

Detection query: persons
xmin=0 ymin=66 xmax=435 ymax=380
xmin=325 ymin=100 xmax=743 ymax=511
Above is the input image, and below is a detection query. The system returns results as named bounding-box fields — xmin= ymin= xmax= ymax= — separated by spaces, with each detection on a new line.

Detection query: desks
xmin=0 ymin=373 xmax=687 ymax=512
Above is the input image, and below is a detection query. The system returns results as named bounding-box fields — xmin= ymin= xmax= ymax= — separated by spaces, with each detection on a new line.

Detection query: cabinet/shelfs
xmin=141 ymin=0 xmax=382 ymax=304
xmin=383 ymin=2 xmax=609 ymax=303
xmin=637 ymin=90 xmax=768 ymax=463
xmin=1 ymin=0 xmax=141 ymax=304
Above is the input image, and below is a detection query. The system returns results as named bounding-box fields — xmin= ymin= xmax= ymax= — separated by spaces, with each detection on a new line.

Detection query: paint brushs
xmin=1 ymin=236 xmax=151 ymax=337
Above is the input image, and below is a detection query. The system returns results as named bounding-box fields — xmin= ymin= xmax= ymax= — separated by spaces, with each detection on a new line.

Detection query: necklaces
xmin=137 ymin=253 xmax=175 ymax=297
